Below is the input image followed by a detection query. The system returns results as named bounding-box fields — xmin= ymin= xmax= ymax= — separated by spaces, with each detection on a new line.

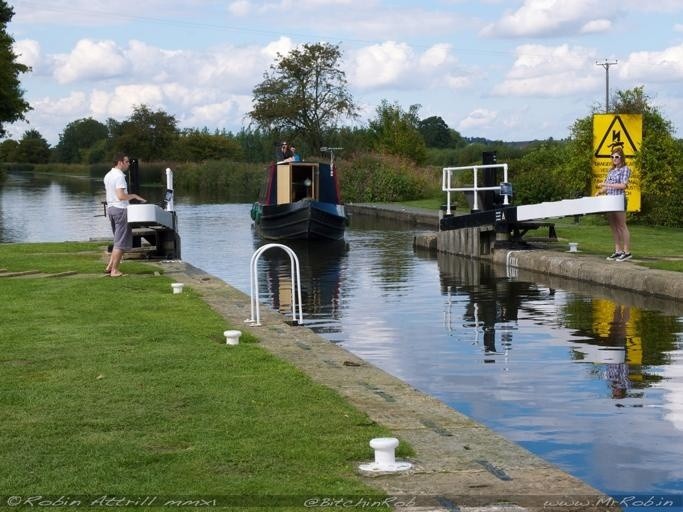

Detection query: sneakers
xmin=606 ymin=251 xmax=625 ymax=261
xmin=616 ymin=252 xmax=633 ymax=262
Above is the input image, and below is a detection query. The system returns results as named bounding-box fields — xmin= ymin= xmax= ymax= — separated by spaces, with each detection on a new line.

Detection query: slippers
xmin=103 ymin=268 xmax=111 ymax=273
xmin=111 ymin=272 xmax=128 ymax=277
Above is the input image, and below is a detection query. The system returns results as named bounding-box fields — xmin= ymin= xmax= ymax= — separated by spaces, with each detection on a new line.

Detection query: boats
xmin=250 ymin=146 xmax=349 ymax=244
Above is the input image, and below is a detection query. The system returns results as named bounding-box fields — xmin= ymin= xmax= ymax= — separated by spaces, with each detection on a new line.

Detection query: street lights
xmin=594 ymin=58 xmax=614 ymax=114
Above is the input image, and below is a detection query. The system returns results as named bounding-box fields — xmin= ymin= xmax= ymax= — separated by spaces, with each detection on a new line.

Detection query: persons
xmin=590 ymin=148 xmax=633 ymax=263
xmin=279 ymin=141 xmax=294 ymax=165
xmin=289 ymin=145 xmax=299 ymax=163
xmin=99 ymin=153 xmax=147 ymax=277
xmin=606 ymin=300 xmax=633 ymax=401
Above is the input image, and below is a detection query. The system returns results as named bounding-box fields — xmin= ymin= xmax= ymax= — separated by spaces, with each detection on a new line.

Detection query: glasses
xmin=610 ymin=155 xmax=622 ymax=159
